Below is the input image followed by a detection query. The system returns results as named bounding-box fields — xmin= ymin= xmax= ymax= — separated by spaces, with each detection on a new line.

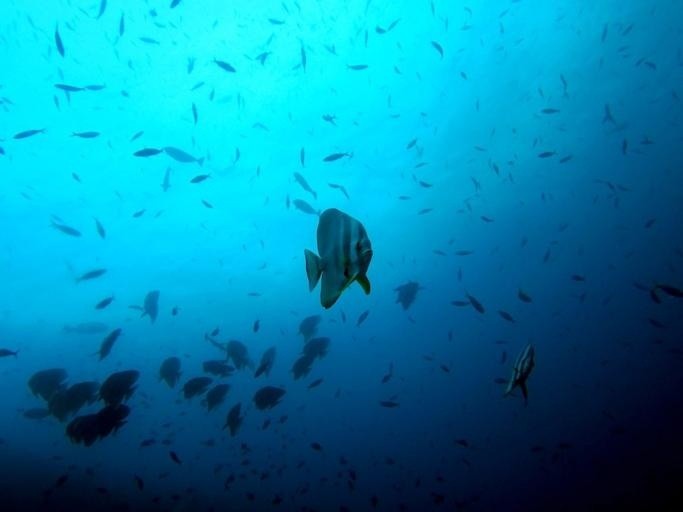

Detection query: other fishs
xmin=0 ymin=0 xmax=683 ymax=512
